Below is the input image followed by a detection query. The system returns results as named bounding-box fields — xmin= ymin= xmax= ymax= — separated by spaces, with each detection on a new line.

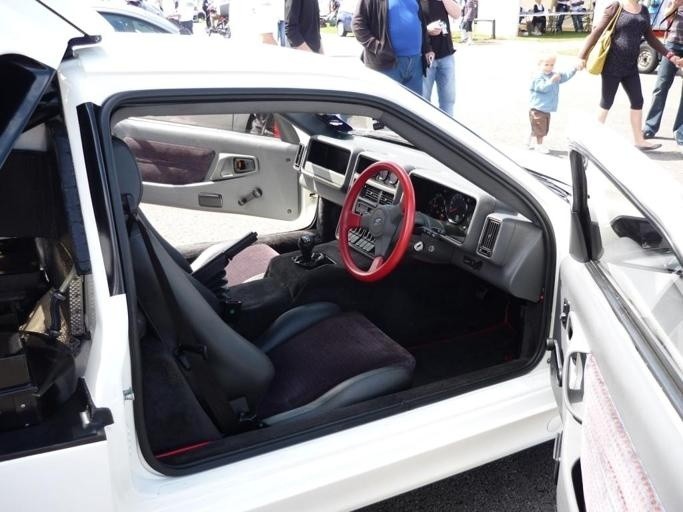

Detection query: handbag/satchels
xmin=586 ymin=4 xmax=623 ymax=75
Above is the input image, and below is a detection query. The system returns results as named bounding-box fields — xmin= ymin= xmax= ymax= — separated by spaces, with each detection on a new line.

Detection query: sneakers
xmin=634 ymin=143 xmax=661 ymax=150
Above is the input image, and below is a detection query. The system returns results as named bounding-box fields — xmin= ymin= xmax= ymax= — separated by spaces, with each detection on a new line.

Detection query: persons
xmin=329 ymin=0 xmax=340 ymax=27
xmin=458 ymin=0 xmax=474 ymax=43
xmin=351 ymin=0 xmax=456 ymax=116
xmin=577 ymin=0 xmax=683 ymax=150
xmin=284 ymin=0 xmax=324 ymax=54
xmin=529 ymin=54 xmax=586 ymax=154
xmin=531 ymin=0 xmax=546 ymax=36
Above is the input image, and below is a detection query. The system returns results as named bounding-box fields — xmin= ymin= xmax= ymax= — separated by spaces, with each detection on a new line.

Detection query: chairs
xmin=2 ymin=326 xmax=79 ymax=430
xmin=2 ymin=236 xmax=48 ymax=288
xmin=108 ymin=136 xmax=416 ymax=456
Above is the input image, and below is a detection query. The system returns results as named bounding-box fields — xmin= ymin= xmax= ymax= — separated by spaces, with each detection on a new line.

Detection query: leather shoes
xmin=673 ymin=130 xmax=683 ymax=145
xmin=644 ymin=130 xmax=655 ymax=138
